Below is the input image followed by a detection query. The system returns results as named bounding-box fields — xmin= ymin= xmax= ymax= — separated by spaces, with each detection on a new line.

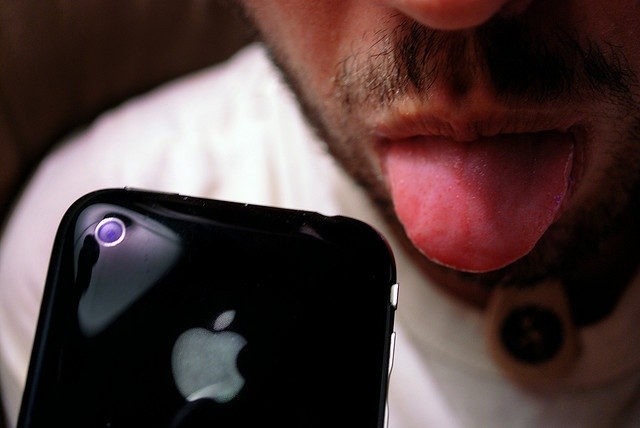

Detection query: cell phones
xmin=20 ymin=187 xmax=400 ymax=425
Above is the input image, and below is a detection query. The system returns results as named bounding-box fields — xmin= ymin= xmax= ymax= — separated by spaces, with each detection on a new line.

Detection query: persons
xmin=0 ymin=2 xmax=640 ymax=425
